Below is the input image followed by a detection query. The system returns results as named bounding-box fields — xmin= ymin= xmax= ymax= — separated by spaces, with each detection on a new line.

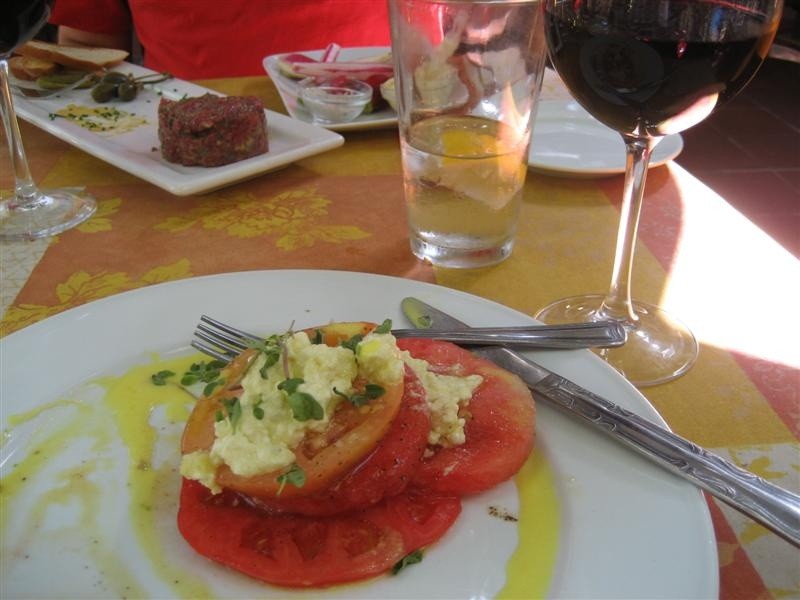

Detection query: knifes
xmin=401 ymin=296 xmax=800 ymax=550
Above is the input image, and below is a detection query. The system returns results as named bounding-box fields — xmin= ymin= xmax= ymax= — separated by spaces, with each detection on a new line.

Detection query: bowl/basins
xmin=289 ymin=76 xmax=373 ymax=124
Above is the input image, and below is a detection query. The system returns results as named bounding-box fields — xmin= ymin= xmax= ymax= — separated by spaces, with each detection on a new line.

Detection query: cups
xmin=386 ymin=1 xmax=546 ymax=268
xmin=1 ymin=55 xmax=345 ymax=197
xmin=1 ymin=0 xmax=97 ymax=242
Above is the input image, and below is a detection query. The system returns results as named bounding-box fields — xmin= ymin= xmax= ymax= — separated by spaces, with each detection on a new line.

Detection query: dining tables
xmin=0 ymin=75 xmax=800 ymax=599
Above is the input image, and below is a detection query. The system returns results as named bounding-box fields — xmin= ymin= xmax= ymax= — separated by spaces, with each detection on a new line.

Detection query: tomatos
xmin=177 ymin=322 xmax=537 ymax=586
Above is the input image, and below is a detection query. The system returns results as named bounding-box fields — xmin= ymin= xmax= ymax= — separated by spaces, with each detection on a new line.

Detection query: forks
xmin=191 ymin=316 xmax=627 ymax=365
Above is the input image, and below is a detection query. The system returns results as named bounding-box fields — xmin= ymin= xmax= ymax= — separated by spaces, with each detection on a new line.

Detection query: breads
xmin=8 ymin=42 xmax=129 ymax=81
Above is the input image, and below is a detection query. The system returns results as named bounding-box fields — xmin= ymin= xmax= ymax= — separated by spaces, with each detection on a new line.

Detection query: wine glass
xmin=536 ymin=1 xmax=784 ymax=388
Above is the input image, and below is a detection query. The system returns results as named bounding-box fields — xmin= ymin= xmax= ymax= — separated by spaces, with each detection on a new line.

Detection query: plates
xmin=263 ymin=46 xmax=469 ymax=132
xmin=471 ymin=88 xmax=683 ymax=178
xmin=0 ymin=269 xmax=719 ymax=600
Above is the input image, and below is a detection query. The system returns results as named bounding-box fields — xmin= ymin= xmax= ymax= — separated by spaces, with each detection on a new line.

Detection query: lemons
xmin=419 ymin=131 xmax=527 ymax=210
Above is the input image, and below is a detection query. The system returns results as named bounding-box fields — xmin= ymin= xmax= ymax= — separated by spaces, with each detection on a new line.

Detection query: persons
xmin=46 ymin=1 xmax=457 ymax=83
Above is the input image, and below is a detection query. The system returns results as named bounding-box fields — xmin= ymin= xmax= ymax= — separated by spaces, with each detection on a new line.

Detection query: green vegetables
xmin=151 ymin=317 xmax=423 ymax=577
